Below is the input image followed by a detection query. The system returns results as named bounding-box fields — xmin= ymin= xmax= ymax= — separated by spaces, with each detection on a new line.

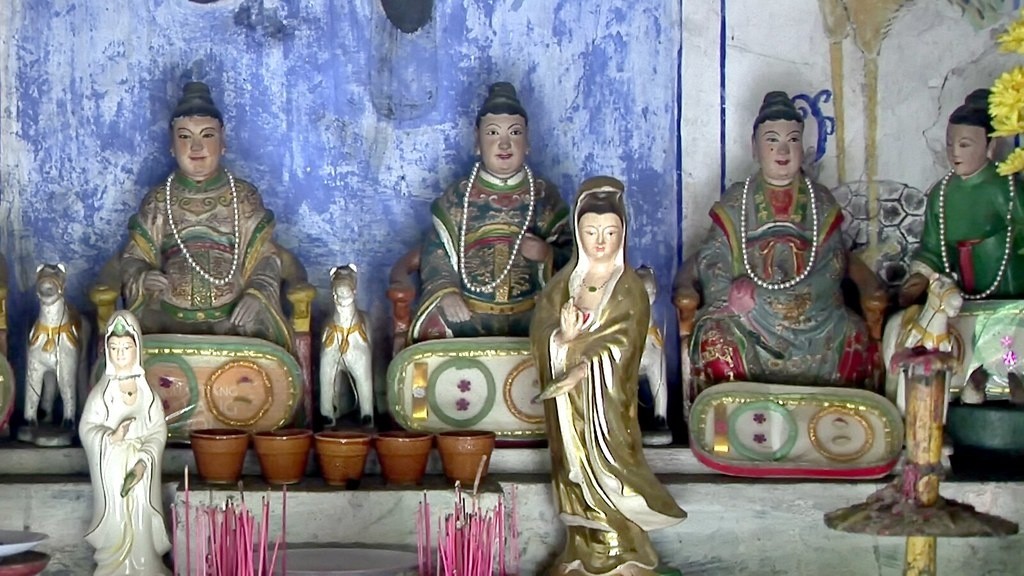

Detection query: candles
xmin=891 ymin=345 xmax=960 ymax=498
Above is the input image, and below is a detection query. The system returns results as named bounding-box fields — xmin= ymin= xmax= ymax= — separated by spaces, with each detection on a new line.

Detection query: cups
xmin=314 ymin=431 xmax=372 ymax=487
xmin=435 ymin=430 xmax=496 ymax=484
xmin=373 ymin=431 xmax=434 ymax=487
xmin=251 ymin=429 xmax=313 ymax=485
xmin=188 ymin=428 xmax=250 ymax=484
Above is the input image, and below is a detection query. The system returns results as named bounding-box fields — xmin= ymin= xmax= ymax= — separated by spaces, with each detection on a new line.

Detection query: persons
xmin=120 ymin=81 xmax=296 ymax=355
xmin=407 ymin=80 xmax=572 ymax=345
xmin=79 ymin=311 xmax=172 ymax=576
xmin=690 ymin=90 xmax=877 ymax=384
xmin=531 ymin=176 xmax=688 ymax=576
xmin=902 ymin=88 xmax=1024 ymax=301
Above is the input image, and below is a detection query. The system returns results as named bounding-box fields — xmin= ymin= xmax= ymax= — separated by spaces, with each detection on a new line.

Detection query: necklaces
xmin=166 ymin=168 xmax=243 ymax=285
xmin=741 ymin=177 xmax=818 ymax=291
xmin=583 ymin=278 xmax=613 ymax=292
xmin=120 ymin=390 xmax=137 ymax=397
xmin=939 ymin=162 xmax=1013 ymax=299
xmin=460 ymin=162 xmax=535 ymax=292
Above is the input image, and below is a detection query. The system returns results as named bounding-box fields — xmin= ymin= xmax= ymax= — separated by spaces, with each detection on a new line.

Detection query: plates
xmin=0 ymin=530 xmax=50 ymax=557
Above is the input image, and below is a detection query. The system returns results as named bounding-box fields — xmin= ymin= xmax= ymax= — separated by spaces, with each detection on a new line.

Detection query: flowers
xmin=987 ymin=4 xmax=1024 ymax=177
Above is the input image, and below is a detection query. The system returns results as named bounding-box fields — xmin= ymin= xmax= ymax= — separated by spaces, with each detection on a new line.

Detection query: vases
xmin=189 ymin=427 xmax=495 ymax=487
